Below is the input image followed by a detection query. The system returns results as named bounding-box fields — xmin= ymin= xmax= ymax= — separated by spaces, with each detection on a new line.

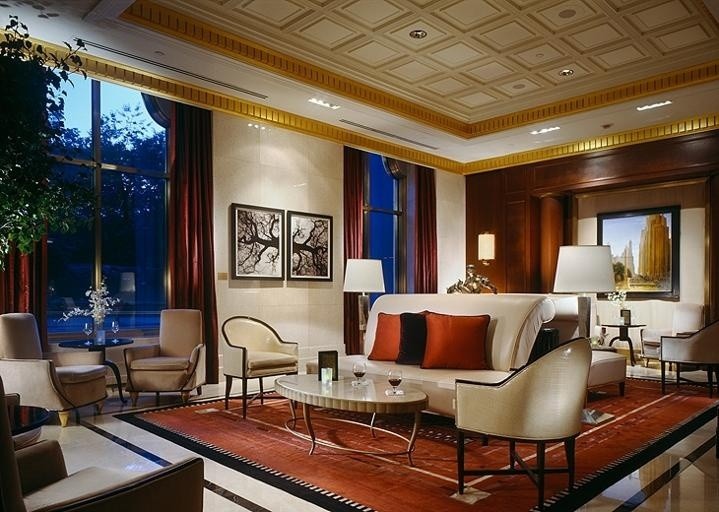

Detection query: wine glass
xmin=111 ymin=321 xmax=120 ymax=343
xmin=353 ymin=360 xmax=367 ymax=386
xmin=82 ymin=322 xmax=93 ymax=346
xmin=389 ymin=371 xmax=403 ymax=397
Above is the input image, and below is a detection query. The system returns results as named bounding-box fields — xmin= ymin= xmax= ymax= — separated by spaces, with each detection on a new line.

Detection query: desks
xmin=600 ymin=324 xmax=645 ymax=366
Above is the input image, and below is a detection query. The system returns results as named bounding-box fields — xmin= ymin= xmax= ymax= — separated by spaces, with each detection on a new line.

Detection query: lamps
xmin=478 ymin=231 xmax=496 ymax=265
xmin=342 ymin=259 xmax=385 ymax=331
xmin=553 ymin=245 xmax=615 ymax=337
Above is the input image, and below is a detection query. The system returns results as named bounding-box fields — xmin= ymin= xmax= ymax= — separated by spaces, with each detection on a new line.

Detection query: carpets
xmin=113 ymin=377 xmax=719 ymax=512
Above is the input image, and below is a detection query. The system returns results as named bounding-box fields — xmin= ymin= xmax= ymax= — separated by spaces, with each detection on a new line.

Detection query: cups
xmin=321 ymin=368 xmax=333 ymax=386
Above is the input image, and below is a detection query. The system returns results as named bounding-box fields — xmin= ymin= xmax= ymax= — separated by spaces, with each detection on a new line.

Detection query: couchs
xmin=541 ymin=295 xmax=626 ymax=401
xmin=306 ymin=294 xmax=557 ymax=446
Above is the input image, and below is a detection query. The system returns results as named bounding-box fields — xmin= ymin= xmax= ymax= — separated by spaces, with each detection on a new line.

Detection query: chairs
xmin=220 ymin=316 xmax=298 ymax=419
xmin=661 ymin=320 xmax=719 ymax=398
xmin=454 ymin=337 xmax=592 ymax=508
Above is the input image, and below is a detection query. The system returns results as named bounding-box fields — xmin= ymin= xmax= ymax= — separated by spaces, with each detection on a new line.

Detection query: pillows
xmin=420 ymin=311 xmax=490 ymax=370
xmin=395 ymin=313 xmax=427 ymax=364
xmin=367 ymin=312 xmax=401 ymax=361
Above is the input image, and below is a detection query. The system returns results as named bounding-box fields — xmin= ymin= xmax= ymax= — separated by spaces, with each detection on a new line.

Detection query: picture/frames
xmin=286 ymin=210 xmax=333 ymax=282
xmin=596 ymin=204 xmax=680 ymax=301
xmin=231 ymin=203 xmax=285 ymax=280
xmin=318 ymin=350 xmax=338 ymax=381
xmin=620 ymin=310 xmax=631 ymax=324
